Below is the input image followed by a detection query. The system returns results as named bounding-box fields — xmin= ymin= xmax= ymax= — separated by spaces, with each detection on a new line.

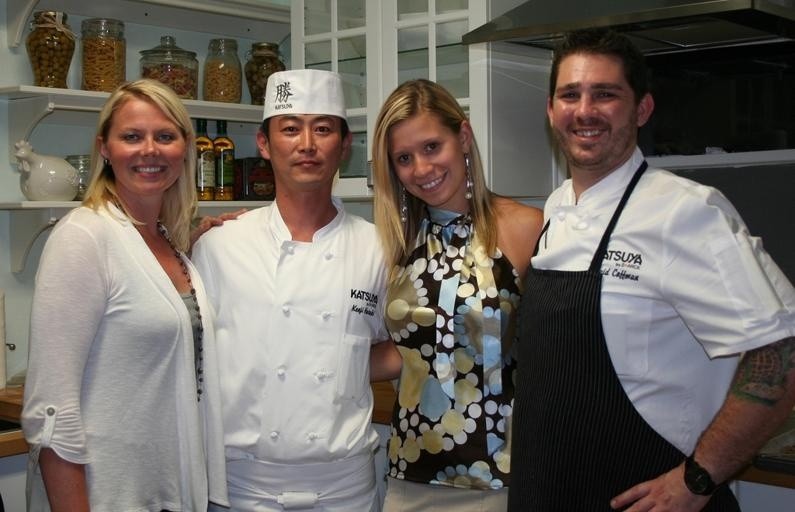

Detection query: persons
xmin=23 ymin=78 xmax=247 ymax=512
xmin=502 ymin=27 xmax=795 ymax=512
xmin=369 ymin=78 xmax=543 ymax=512
xmin=188 ymin=68 xmax=389 ymax=512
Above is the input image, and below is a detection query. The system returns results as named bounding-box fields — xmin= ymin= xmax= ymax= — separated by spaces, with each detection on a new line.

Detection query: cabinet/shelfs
xmin=3 ymin=2 xmax=292 ymax=213
xmin=290 ymin=0 xmax=556 ymax=200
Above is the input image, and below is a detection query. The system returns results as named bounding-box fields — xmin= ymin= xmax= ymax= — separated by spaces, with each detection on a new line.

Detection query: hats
xmin=263 ymin=68 xmax=347 ymax=121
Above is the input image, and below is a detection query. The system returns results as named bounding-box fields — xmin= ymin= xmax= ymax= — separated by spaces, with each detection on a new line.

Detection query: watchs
xmin=683 ymin=453 xmax=723 ymax=496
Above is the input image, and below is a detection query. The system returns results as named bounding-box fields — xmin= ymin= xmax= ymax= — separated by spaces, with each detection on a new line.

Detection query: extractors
xmin=464 ymin=0 xmax=792 ymax=65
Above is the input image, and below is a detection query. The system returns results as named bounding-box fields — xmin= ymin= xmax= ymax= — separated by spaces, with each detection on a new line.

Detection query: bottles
xmin=26 ymin=9 xmax=284 ymax=106
xmin=193 ymin=118 xmax=237 ymax=199
xmin=62 ymin=154 xmax=95 ymax=202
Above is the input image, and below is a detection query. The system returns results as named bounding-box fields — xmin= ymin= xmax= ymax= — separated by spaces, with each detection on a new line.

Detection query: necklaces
xmin=112 ymin=196 xmax=204 ymax=401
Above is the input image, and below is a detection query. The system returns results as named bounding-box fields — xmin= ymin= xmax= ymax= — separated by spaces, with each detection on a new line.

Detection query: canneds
xmin=244 ymin=42 xmax=286 ymax=106
xmin=80 ymin=18 xmax=125 ymax=93
xmin=25 ymin=11 xmax=74 ymax=89
xmin=66 ymin=154 xmax=93 ymax=201
